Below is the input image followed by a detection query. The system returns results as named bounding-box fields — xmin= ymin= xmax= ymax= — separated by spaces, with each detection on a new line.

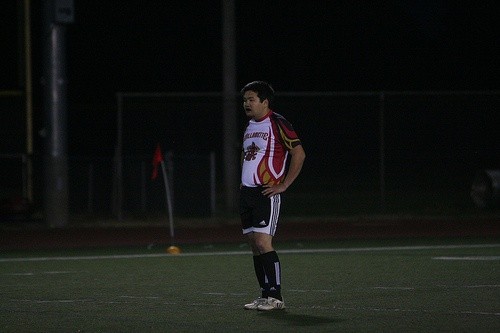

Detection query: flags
xmin=152 ymin=146 xmax=163 ymax=180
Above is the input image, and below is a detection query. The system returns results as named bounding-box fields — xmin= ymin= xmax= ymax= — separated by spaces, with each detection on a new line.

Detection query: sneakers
xmin=245 ymin=296 xmax=285 ymax=310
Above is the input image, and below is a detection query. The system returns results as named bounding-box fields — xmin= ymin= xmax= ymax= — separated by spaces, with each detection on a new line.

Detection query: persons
xmin=238 ymin=80 xmax=306 ymax=311
xmin=160 ymin=151 xmax=175 ymax=217
